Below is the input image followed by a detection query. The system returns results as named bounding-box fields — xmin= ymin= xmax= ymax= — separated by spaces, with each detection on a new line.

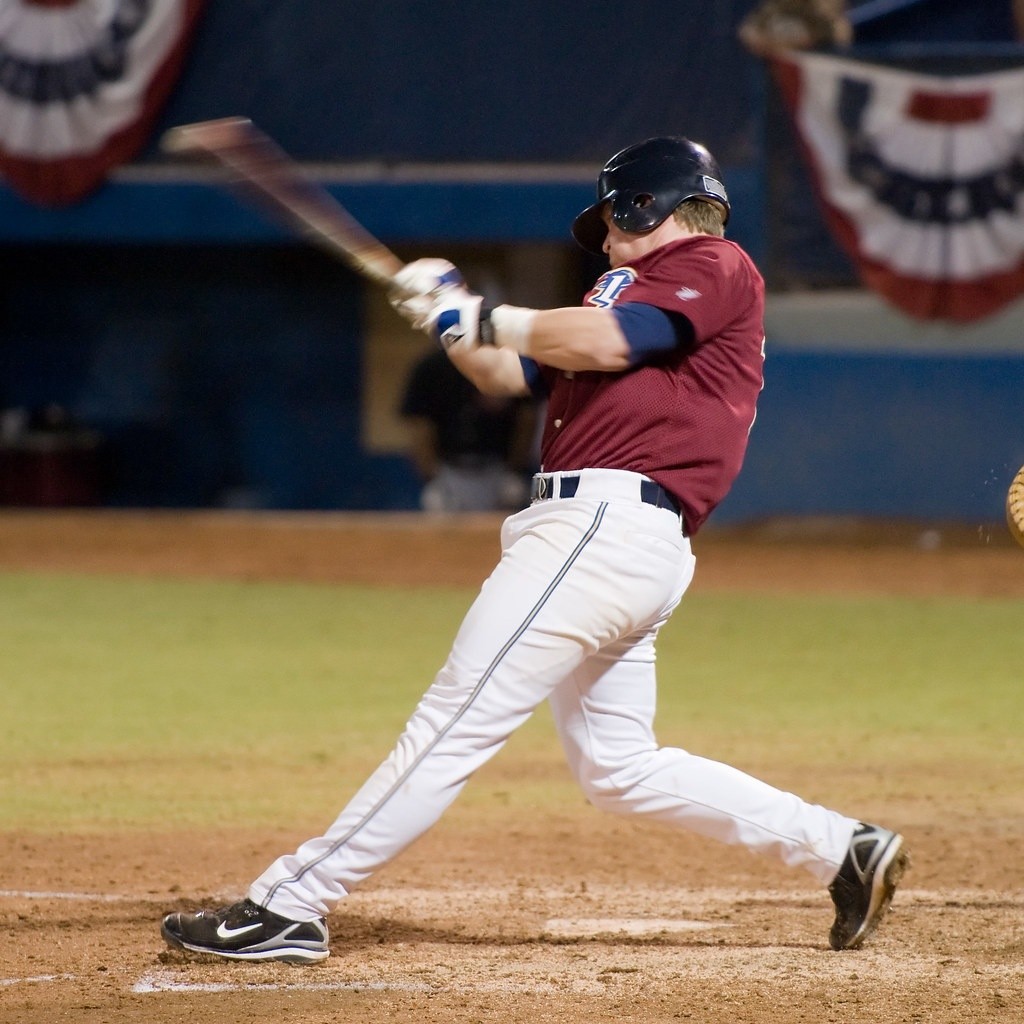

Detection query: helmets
xmin=570 ymin=134 xmax=731 ymax=258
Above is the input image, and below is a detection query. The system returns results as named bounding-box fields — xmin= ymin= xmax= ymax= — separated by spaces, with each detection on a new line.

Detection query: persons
xmin=734 ymin=0 xmax=858 ymax=286
xmin=393 ymin=266 xmax=547 ymax=513
xmin=160 ymin=138 xmax=907 ymax=967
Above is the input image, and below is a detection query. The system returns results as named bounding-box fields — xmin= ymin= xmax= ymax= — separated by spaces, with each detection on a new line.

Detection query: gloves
xmin=384 ymin=257 xmax=484 ymax=349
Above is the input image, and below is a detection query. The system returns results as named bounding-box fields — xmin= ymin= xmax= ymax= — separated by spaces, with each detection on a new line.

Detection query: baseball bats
xmin=176 ymin=113 xmax=404 ymax=290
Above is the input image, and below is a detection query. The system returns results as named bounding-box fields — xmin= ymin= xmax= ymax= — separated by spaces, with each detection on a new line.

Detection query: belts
xmin=529 ymin=476 xmax=682 ymax=515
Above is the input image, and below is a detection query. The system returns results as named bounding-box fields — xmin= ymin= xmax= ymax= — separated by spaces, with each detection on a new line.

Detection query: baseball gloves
xmin=1005 ymin=466 xmax=1024 ymax=549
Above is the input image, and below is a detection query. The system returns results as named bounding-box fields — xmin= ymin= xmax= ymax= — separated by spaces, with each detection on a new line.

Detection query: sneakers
xmin=160 ymin=896 xmax=331 ymax=964
xmin=826 ymin=821 xmax=905 ymax=951
